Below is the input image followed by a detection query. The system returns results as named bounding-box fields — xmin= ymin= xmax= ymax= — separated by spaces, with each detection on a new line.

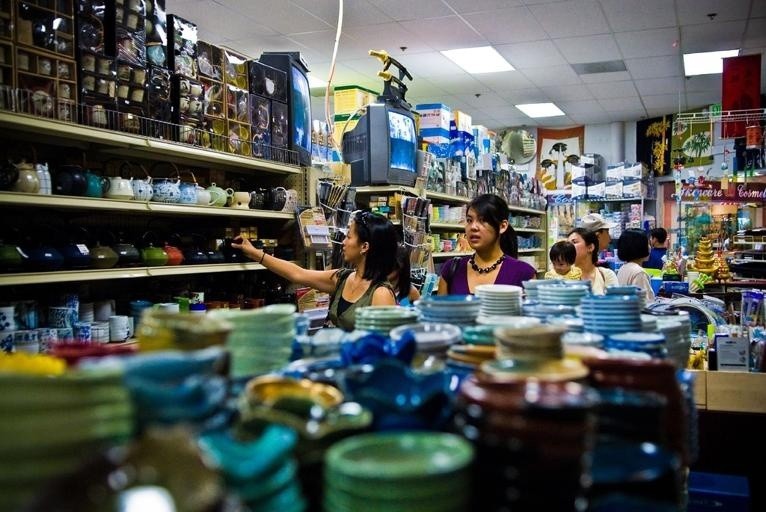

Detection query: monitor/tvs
xmin=342 ymin=103 xmax=418 ymax=187
xmin=258 ymin=54 xmax=312 ymax=167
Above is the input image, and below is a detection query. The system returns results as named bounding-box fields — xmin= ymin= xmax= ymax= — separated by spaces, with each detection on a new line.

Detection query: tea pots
xmin=0 ymin=157 xmax=297 ymax=211
xmin=0 ymin=227 xmax=244 ymax=271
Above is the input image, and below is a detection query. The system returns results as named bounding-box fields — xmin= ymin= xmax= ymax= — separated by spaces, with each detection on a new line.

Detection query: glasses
xmin=355 ymin=210 xmax=370 ymax=242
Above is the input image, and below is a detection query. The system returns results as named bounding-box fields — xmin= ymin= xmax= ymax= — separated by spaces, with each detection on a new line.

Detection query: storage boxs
xmin=569 ymin=153 xmax=648 ymax=199
xmin=332 ymin=84 xmax=381 ymax=149
xmin=415 ymin=104 xmax=497 ymax=163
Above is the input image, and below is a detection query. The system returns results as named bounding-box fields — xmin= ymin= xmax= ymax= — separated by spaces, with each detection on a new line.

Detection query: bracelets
xmin=259 ymin=252 xmax=266 ymax=264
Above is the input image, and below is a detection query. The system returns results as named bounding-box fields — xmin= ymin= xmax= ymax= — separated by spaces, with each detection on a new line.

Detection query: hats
xmin=581 ymin=213 xmax=619 ymax=231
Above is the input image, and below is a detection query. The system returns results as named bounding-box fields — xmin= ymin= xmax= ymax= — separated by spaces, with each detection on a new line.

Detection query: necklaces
xmin=348 ymin=271 xmax=368 ymax=294
xmin=469 ymin=252 xmax=506 ymax=274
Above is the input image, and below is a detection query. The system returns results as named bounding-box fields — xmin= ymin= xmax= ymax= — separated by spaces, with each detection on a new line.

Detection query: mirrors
xmin=498 ymin=128 xmax=537 ymax=164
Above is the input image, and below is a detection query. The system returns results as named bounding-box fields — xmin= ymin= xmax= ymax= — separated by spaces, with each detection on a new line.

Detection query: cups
xmin=428 ymin=231 xmax=543 ymax=253
xmin=1 ymin=287 xmax=265 ymax=353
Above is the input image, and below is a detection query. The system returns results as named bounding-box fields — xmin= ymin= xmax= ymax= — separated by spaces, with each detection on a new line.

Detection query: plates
xmin=427 ymin=202 xmax=542 ymax=229
xmin=0 ymin=280 xmax=690 ymax=512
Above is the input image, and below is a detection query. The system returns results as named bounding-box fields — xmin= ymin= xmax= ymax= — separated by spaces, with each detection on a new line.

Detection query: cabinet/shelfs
xmin=572 ymin=196 xmax=655 ymax=264
xmin=348 ymin=183 xmax=549 ymax=280
xmin=0 ymin=104 xmax=303 ymax=356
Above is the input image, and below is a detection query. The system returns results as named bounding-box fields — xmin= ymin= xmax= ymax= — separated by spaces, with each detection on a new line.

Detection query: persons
xmin=568 ymin=227 xmax=619 ymax=295
xmin=437 ymin=193 xmax=539 ymax=300
xmin=230 ymin=210 xmax=395 ymax=333
xmin=581 ymin=213 xmax=617 ymax=252
xmin=617 ymin=227 xmax=657 ymax=304
xmin=385 ymin=246 xmax=421 ymax=307
xmin=544 ymin=240 xmax=583 ymax=280
xmin=642 ymin=226 xmax=669 ymax=270
